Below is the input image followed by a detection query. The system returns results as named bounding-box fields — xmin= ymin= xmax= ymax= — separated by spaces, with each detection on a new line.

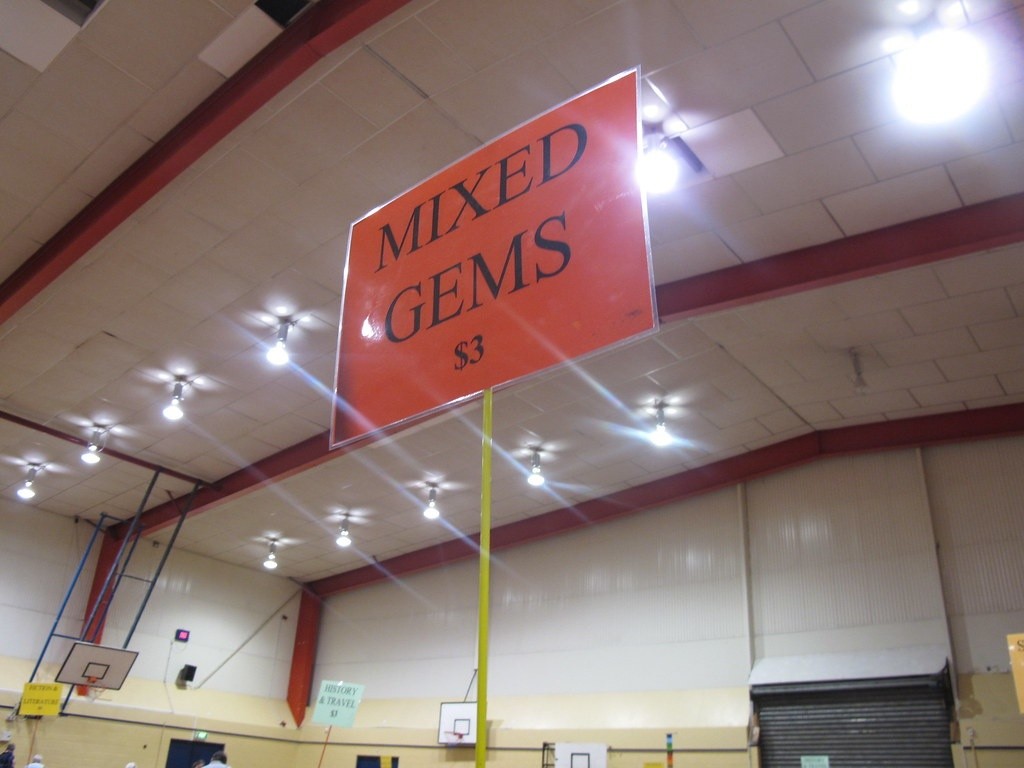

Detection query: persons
xmin=201 ymin=751 xmax=229 ymax=768
xmin=192 ymin=759 xmax=205 ymax=768
xmin=25 ymin=754 xmax=44 ymax=768
xmin=0 ymin=743 xmax=16 ymax=768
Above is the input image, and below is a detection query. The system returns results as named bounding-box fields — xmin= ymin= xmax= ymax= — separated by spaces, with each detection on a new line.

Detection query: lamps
xmin=651 ymin=409 xmax=671 ymax=447
xmin=162 ymin=383 xmax=184 ymax=421
xmin=423 ymin=489 xmax=439 ymax=519
xmin=81 ymin=431 xmax=102 ymax=464
xmin=336 ymin=519 xmax=351 ymax=547
xmin=267 ymin=324 xmax=289 ymax=367
xmin=264 ymin=543 xmax=277 ymax=569
xmin=848 ymin=350 xmax=867 ymax=393
xmin=527 ymin=454 xmax=545 ymax=486
xmin=17 ymin=468 xmax=37 ymax=499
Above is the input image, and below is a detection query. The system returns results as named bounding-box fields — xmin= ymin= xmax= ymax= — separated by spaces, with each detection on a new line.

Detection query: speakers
xmin=181 ymin=665 xmax=197 ymax=682
